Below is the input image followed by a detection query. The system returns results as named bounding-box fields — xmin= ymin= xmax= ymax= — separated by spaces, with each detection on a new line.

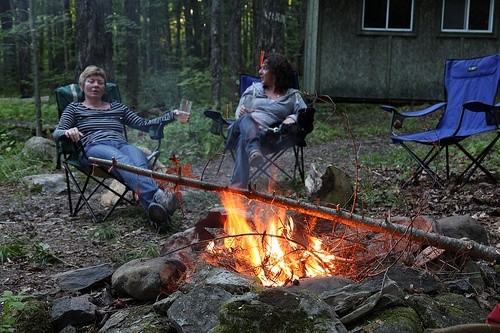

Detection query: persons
xmin=53 ymin=65 xmax=190 ymax=232
xmin=224 ymin=53 xmax=307 ymax=189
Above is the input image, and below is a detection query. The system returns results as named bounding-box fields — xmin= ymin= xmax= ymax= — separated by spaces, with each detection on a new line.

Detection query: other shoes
xmin=148 ymin=203 xmax=171 ymax=233
xmin=155 ymin=190 xmax=183 ymax=216
xmin=249 ymin=150 xmax=264 ymax=167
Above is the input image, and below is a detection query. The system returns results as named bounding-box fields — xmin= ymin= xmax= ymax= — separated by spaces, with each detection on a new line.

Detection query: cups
xmin=177 ymin=99 xmax=193 ymax=123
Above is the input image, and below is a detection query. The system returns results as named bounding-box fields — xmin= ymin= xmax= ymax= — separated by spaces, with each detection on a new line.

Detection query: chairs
xmin=379 ymin=52 xmax=500 ymax=189
xmin=55 ymin=83 xmax=140 ymax=224
xmin=200 ymin=74 xmax=317 ymax=187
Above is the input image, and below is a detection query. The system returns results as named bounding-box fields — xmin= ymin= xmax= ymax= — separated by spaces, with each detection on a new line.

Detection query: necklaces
xmin=271 ymin=90 xmax=280 ymax=98
